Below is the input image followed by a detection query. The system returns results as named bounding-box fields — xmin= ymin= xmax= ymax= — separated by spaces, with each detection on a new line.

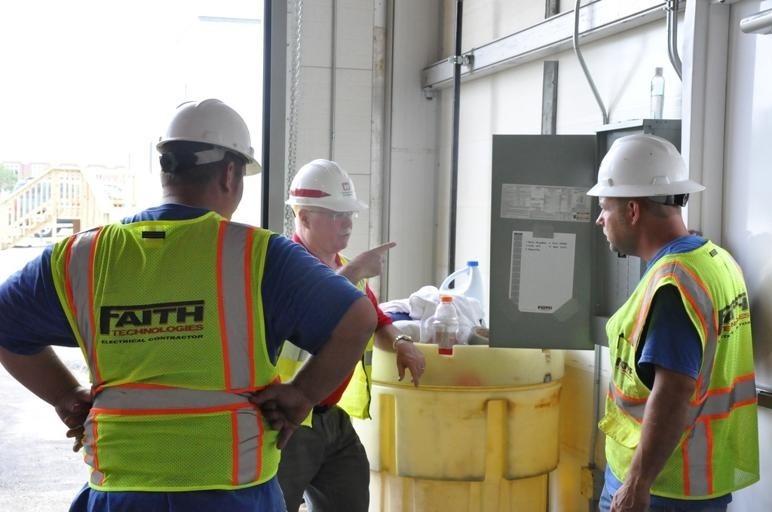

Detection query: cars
xmin=9 ymin=177 xmax=75 ymax=239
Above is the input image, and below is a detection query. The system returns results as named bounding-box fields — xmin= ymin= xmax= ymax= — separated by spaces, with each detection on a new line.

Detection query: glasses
xmin=330 ymin=212 xmax=359 ymax=224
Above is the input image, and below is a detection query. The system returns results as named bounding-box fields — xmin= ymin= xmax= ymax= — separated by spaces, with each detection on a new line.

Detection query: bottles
xmin=431 ymin=294 xmax=459 ymax=355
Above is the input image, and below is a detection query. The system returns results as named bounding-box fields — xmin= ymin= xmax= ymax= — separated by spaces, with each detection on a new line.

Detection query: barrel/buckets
xmin=440 ymin=261 xmax=483 ymax=308
xmin=349 ymin=343 xmax=566 ymax=512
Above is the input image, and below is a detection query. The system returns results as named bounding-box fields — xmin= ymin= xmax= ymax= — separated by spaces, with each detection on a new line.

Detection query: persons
xmin=277 ymin=157 xmax=422 ymax=512
xmin=1 ymin=94 xmax=376 ymax=511
xmin=582 ymin=133 xmax=764 ymax=512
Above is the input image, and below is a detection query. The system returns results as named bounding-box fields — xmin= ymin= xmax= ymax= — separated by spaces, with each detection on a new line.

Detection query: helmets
xmin=286 ymin=158 xmax=368 ymax=214
xmin=586 ymin=133 xmax=706 ymax=201
xmin=156 ymin=96 xmax=262 ymax=176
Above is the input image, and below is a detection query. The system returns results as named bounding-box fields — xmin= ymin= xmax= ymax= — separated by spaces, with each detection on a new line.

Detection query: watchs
xmin=391 ymin=333 xmax=414 ymax=354
